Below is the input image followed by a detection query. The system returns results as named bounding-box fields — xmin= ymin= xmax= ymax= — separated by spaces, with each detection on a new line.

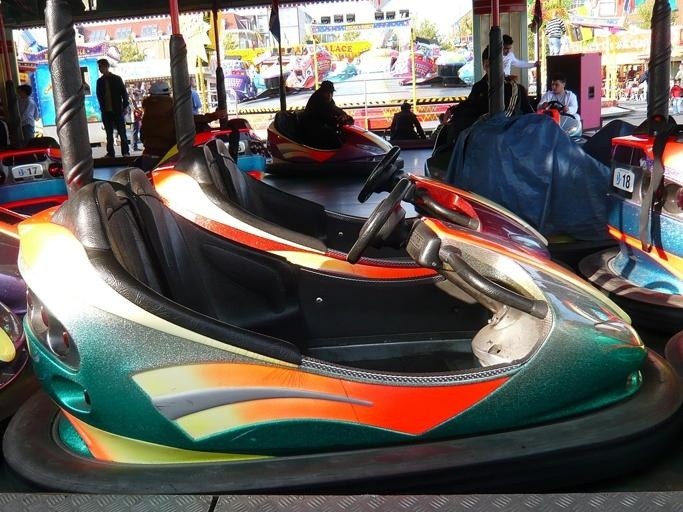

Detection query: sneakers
xmin=102 ymin=147 xmax=143 ymax=157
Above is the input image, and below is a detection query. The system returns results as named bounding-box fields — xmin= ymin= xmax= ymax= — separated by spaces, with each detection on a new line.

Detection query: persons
xmin=96 ymin=58 xmax=129 ymax=159
xmin=17 ymin=84 xmax=39 ymax=139
xmin=670 ymin=79 xmax=683 ymax=98
xmin=389 ymin=103 xmax=426 ymax=140
xmin=536 ymin=71 xmax=578 ymax=115
xmin=139 ymin=80 xmax=226 ymax=160
xmin=297 ymin=79 xmax=353 ymax=149
xmin=189 ymin=76 xmax=204 ymax=115
xmin=502 ymin=33 xmax=540 ymax=82
xmin=674 ymin=62 xmax=682 ymax=88
xmin=545 ymin=10 xmax=566 ymax=55
xmin=432 ymin=44 xmax=528 ymax=169
xmin=631 ymin=62 xmax=649 ymax=118
xmin=625 ymin=67 xmax=636 ymax=83
xmin=125 ymin=82 xmax=151 ymax=152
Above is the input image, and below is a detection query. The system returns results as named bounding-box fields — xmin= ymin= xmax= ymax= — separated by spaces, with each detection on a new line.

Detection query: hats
xmin=148 ymin=82 xmax=171 ymax=95
xmin=319 ymin=81 xmax=335 ymax=91
